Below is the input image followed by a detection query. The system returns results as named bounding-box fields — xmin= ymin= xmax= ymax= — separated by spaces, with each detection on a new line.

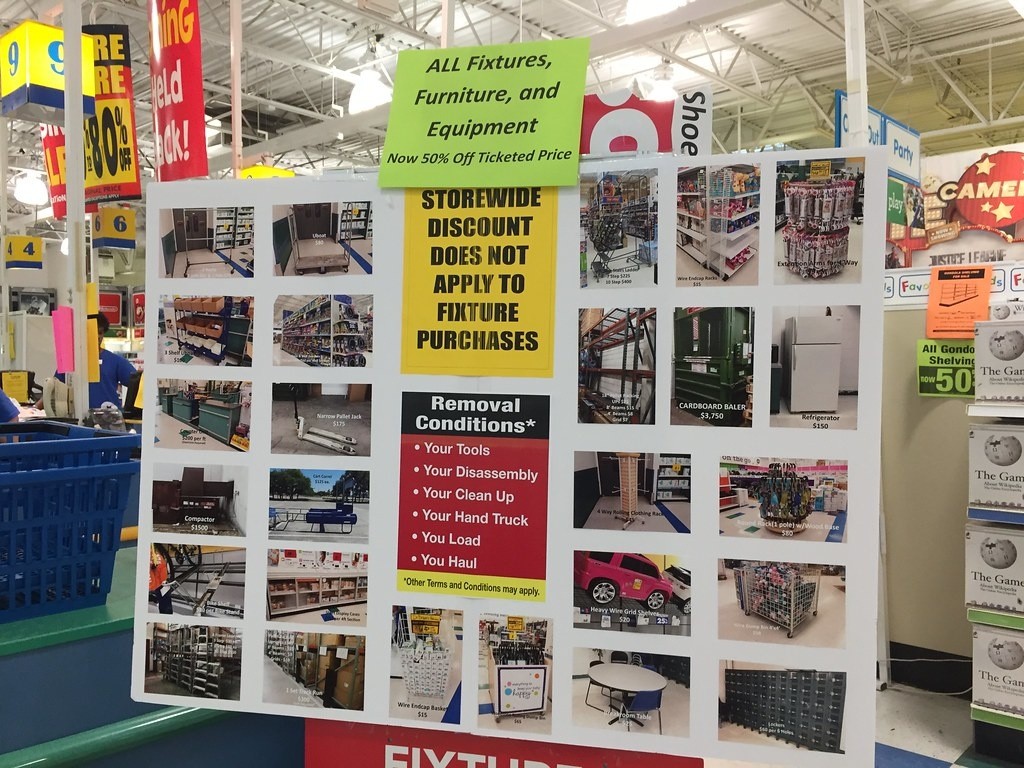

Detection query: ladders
xmin=162 ymin=628 xmax=226 ymax=699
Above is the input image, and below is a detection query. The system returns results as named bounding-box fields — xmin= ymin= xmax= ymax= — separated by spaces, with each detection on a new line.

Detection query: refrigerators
xmin=783 ymin=317 xmax=841 ymax=413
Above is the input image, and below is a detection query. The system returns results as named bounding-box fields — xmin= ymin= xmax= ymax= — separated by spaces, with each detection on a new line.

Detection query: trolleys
xmin=285 ymin=201 xmax=354 ymax=275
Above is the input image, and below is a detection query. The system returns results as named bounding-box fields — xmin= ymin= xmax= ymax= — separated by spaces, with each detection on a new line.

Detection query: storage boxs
xmin=248 ymin=301 xmax=254 ymax=318
xmin=246 ymin=342 xmax=253 ymax=359
xmin=293 ymin=632 xmax=365 ymax=709
xmin=173 ymin=297 xmax=225 ymax=337
xmin=1 ymin=420 xmax=140 ymax=621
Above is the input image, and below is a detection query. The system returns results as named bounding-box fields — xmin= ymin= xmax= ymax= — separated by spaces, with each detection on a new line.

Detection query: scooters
xmin=288 ymin=384 xmax=358 ymax=456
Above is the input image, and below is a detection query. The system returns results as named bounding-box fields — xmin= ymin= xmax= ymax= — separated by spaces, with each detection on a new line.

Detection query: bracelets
xmin=32 ymin=405 xmax=39 ymax=409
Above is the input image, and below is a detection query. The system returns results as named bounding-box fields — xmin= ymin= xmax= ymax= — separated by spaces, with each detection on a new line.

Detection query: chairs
xmin=586 ymin=651 xmax=665 ymax=736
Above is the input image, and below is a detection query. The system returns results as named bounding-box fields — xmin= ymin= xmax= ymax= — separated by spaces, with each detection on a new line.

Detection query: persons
xmin=0 ymin=388 xmax=20 ymax=443
xmin=29 ymin=311 xmax=138 ymax=415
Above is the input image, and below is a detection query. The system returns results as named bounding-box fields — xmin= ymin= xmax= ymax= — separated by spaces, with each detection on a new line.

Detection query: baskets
xmin=0 ymin=419 xmax=142 ymax=624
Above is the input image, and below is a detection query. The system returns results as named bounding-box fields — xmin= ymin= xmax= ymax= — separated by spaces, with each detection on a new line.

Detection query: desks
xmin=588 ymin=663 xmax=667 ymax=726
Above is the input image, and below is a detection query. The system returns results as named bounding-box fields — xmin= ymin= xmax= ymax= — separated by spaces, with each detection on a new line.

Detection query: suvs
xmin=662 ymin=564 xmax=691 ymax=615
xmin=573 ymin=550 xmax=673 ymax=610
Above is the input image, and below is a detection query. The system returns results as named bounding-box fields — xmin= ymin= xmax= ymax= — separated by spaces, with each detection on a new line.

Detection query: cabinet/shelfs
xmin=334 ymin=201 xmax=372 ymax=240
xmin=719 ymin=484 xmax=738 ymax=511
xmin=214 ymin=207 xmax=254 ymax=249
xmin=267 ymin=575 xmax=368 ymax=617
xmin=676 ymin=166 xmax=759 ymax=280
xmin=171 ymin=298 xmax=234 ymax=365
xmin=646 ymin=454 xmax=693 ymax=503
xmin=280 ymin=295 xmax=352 ymax=368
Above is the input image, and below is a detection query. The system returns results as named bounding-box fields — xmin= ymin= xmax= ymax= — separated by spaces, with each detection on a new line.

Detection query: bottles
xmin=334 ymin=340 xmax=348 ymax=354
xmin=332 ymin=356 xmax=345 ymax=367
